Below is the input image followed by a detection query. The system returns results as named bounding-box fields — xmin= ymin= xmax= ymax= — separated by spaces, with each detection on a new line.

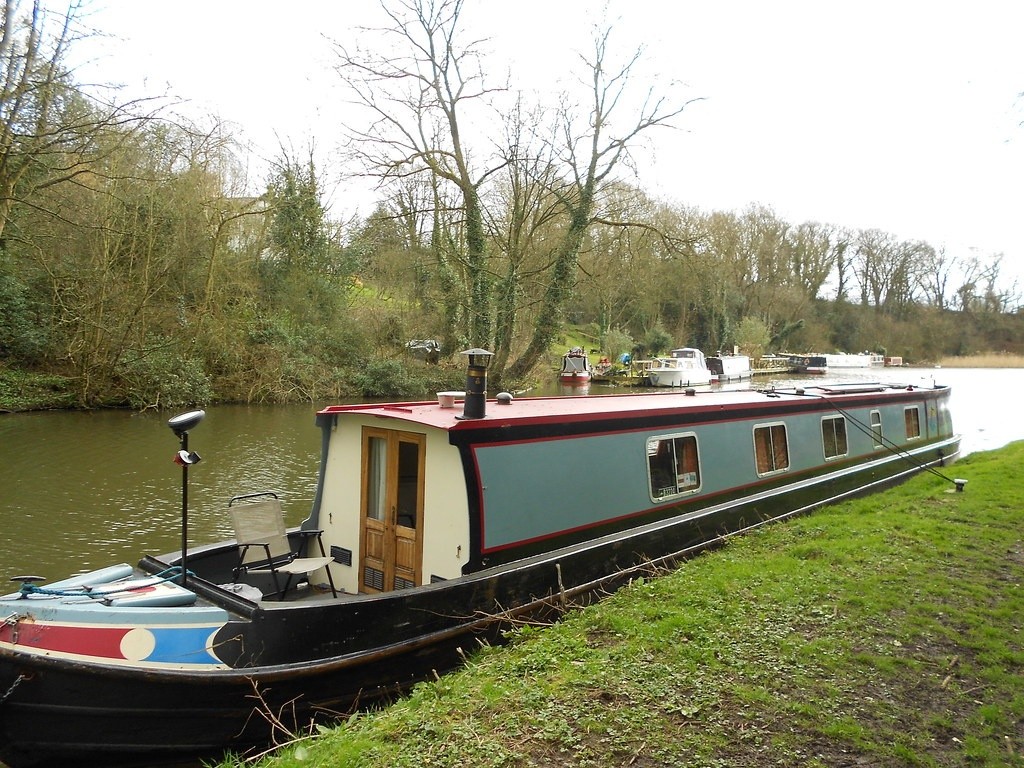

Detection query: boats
xmin=558 ymin=345 xmax=592 ymax=382
xmin=592 ymin=356 xmax=612 ymax=380
xmin=777 ymin=352 xmax=829 ymax=374
xmin=751 ymin=354 xmax=800 ymax=374
xmin=0 ymin=383 xmax=961 ymax=768
xmin=707 ymin=350 xmax=754 ymax=379
xmin=645 ymin=347 xmax=719 ymax=386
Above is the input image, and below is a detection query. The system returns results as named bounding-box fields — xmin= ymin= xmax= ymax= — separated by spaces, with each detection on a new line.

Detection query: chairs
xmin=229 ymin=490 xmax=337 ymax=601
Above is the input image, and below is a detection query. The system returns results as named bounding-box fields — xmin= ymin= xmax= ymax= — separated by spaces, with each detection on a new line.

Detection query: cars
xmin=404 ymin=339 xmax=440 ymax=364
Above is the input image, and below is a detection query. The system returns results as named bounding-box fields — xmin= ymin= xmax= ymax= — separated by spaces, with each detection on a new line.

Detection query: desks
xmin=631 ymin=361 xmax=653 ymax=378
